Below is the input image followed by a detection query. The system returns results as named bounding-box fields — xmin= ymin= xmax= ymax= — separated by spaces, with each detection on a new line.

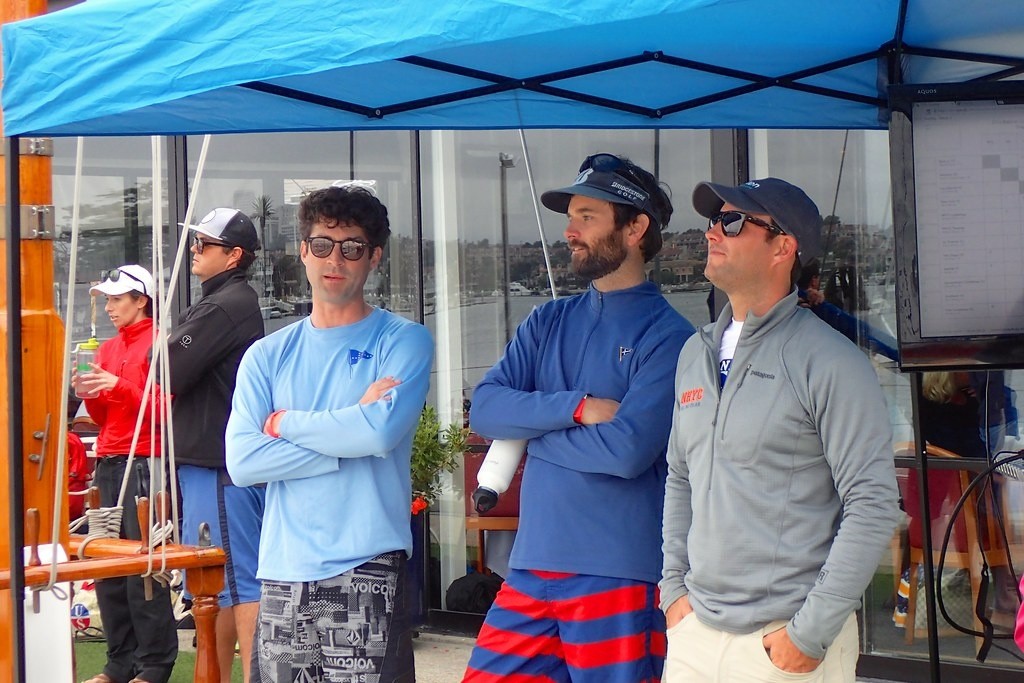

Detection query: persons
xmin=154 ymin=206 xmax=269 ymax=683
xmin=462 ymin=154 xmax=696 ymax=683
xmin=655 ymin=177 xmax=902 ymax=683
xmin=952 ymin=369 xmax=1021 ymax=614
xmin=796 ymin=258 xmax=826 ymax=308
xmin=72 ymin=264 xmax=179 ymax=683
xmin=224 ymin=187 xmax=434 ymax=682
xmin=920 ymin=370 xmax=1000 ymax=496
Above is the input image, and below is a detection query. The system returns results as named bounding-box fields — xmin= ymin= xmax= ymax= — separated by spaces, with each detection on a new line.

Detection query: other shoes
xmin=128 ymin=678 xmax=153 ymax=683
xmin=81 ymin=673 xmax=112 ymax=683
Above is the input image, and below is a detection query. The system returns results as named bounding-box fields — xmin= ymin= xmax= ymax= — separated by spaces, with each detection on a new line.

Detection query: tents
xmin=1 ymin=2 xmax=1022 ymax=682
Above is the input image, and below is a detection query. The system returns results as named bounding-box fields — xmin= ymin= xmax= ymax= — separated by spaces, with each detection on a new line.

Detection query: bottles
xmin=472 ymin=439 xmax=528 ymax=514
xmin=69 ymin=338 xmax=100 ymax=399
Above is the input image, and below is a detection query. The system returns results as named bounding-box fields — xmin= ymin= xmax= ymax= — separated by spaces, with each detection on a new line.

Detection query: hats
xmin=541 ymin=167 xmax=665 ymax=229
xmin=692 ymin=177 xmax=825 ymax=268
xmin=89 ymin=265 xmax=154 ymax=298
xmin=178 ymin=208 xmax=257 ymax=253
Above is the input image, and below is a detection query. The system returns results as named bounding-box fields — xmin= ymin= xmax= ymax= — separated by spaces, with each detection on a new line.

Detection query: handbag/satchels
xmin=891 ymin=560 xmax=995 ymax=639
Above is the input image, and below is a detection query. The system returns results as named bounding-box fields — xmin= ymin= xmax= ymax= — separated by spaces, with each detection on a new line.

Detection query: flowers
xmin=410 ymin=404 xmax=475 ymax=515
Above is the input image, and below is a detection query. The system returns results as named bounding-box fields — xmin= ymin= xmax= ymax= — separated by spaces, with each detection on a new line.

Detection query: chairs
xmin=462 ymin=432 xmax=528 ymax=573
xmin=892 ymin=440 xmax=1024 ymax=657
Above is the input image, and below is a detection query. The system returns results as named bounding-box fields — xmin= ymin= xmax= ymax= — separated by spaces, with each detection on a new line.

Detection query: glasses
xmin=101 ymin=269 xmax=147 ymax=295
xmin=193 ymin=237 xmax=234 ymax=254
xmin=708 ymin=211 xmax=786 ymax=238
xmin=305 ymin=237 xmax=374 ymax=261
xmin=578 ymin=153 xmax=651 ymax=194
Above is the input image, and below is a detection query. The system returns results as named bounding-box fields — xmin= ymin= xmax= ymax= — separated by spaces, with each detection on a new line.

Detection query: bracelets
xmin=573 ymin=395 xmax=587 ymax=423
xmin=266 ymin=410 xmax=286 ymax=440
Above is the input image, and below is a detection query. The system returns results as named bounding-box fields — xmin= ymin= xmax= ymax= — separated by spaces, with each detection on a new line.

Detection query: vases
xmin=408 ymin=508 xmax=431 ymax=630
